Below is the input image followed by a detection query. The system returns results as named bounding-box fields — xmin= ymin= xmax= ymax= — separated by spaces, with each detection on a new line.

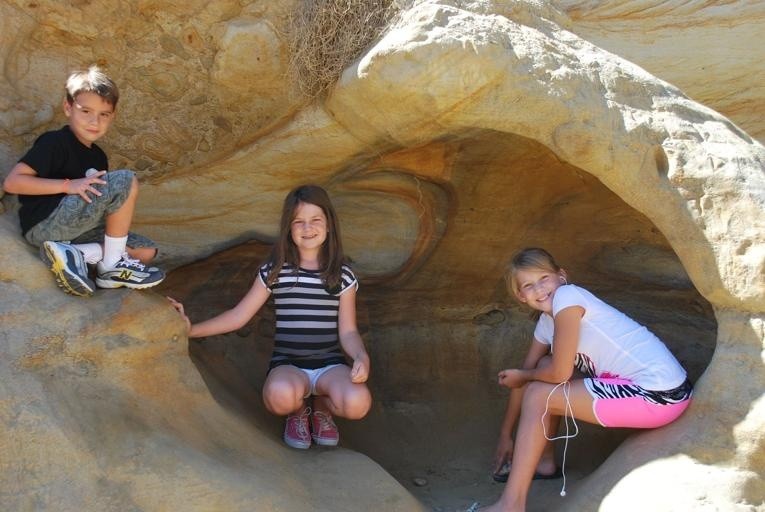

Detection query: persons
xmin=464 ymin=248 xmax=694 ymax=512
xmin=2 ymin=67 xmax=166 ymax=297
xmin=167 ymin=185 xmax=373 ymax=449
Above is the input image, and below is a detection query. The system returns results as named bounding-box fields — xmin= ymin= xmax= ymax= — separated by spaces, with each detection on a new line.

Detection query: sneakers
xmin=39 ymin=241 xmax=96 ymax=298
xmin=95 ymin=253 xmax=167 ymax=290
xmin=284 ymin=407 xmax=339 ymax=449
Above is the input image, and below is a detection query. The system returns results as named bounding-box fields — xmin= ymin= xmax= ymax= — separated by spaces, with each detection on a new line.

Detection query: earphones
xmin=560 ymin=489 xmax=566 ymax=497
xmin=560 ymin=274 xmax=566 ymax=280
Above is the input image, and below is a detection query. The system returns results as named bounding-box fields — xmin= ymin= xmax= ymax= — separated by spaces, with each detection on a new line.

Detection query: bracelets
xmin=63 ymin=178 xmax=71 ymax=194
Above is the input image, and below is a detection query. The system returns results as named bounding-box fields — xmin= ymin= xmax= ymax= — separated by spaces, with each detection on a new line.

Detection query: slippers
xmin=494 ymin=463 xmax=563 ymax=483
